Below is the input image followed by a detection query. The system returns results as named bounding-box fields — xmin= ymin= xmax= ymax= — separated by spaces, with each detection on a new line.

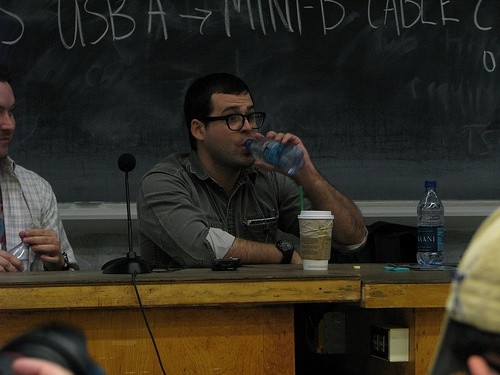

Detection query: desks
xmin=0 ymin=263 xmax=452 ymax=375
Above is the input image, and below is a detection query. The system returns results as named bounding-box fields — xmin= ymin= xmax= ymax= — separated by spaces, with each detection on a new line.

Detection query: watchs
xmin=275 ymin=239 xmax=295 ymax=266
xmin=62 ymin=253 xmax=70 ymax=270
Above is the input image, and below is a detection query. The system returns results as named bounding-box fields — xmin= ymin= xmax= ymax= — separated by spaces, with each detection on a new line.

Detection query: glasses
xmin=206 ymin=112 xmax=265 ymax=131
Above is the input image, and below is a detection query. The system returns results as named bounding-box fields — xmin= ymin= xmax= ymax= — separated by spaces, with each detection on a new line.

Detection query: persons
xmin=423 ymin=205 xmax=499 ymax=374
xmin=0 ymin=356 xmax=73 ymax=374
xmin=1 ymin=77 xmax=85 ymax=272
xmin=135 ymin=71 xmax=368 ymax=264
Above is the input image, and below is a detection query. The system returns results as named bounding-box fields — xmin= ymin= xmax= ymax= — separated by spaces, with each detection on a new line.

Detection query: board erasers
xmin=74 ymin=201 xmax=107 ymax=210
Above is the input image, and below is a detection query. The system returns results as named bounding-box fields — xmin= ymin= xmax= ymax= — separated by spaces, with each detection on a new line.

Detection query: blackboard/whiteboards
xmin=0 ymin=0 xmax=500 ymax=217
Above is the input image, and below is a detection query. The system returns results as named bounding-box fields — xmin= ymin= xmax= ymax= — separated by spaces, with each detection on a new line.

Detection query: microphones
xmin=100 ymin=152 xmax=154 ymax=274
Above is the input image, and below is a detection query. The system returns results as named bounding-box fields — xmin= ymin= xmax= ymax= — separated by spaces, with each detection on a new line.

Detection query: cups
xmin=298 ymin=210 xmax=334 ymax=270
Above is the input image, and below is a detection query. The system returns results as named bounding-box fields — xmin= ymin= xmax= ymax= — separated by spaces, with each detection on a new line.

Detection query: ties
xmin=0 ymin=186 xmax=7 ymax=251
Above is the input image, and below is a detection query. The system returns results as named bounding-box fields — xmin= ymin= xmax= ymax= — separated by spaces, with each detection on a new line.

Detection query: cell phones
xmin=212 ymin=257 xmax=240 ymax=268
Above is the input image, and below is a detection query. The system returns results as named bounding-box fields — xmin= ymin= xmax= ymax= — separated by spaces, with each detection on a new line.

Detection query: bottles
xmin=8 ymin=241 xmax=30 ymax=273
xmin=429 ymin=206 xmax=500 ymax=375
xmin=246 ymin=138 xmax=305 ymax=174
xmin=417 ymin=180 xmax=444 ymax=268
